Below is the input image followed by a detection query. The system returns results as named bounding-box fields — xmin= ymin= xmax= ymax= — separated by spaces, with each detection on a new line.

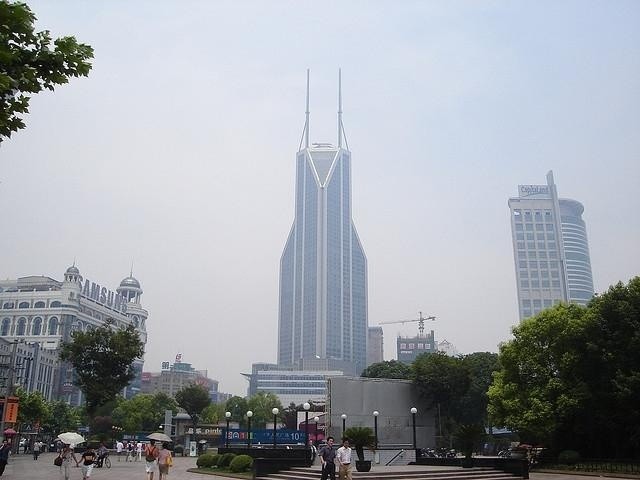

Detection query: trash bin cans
xmin=49 ymin=442 xmax=57 ymax=452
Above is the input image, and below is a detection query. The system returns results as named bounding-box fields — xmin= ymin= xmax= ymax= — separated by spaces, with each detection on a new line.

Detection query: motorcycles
xmin=417 ymin=447 xmax=456 ymax=458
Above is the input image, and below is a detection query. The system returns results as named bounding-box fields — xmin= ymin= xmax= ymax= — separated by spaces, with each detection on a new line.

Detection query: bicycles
xmin=91 ymin=452 xmax=112 ymax=468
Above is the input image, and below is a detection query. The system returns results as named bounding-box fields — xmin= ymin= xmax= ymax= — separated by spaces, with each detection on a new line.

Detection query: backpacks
xmin=146 ymin=446 xmax=156 ymax=462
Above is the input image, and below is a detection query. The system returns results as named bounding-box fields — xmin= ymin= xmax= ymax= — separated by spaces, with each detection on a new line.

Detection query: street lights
xmin=303 ymin=403 xmax=311 ymax=445
xmin=272 ymin=408 xmax=279 ymax=447
xmin=341 ymin=413 xmax=348 ymax=443
xmin=225 ymin=411 xmax=231 ymax=448
xmin=410 ymin=407 xmax=417 ymax=449
xmin=314 ymin=416 xmax=320 ymax=443
xmin=112 ymin=425 xmax=123 ymax=449
xmin=372 ymin=410 xmax=380 ymax=448
xmin=246 ymin=411 xmax=253 ymax=448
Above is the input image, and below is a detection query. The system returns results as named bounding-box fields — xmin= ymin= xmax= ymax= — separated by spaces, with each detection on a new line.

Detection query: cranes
xmin=378 ymin=311 xmax=436 ymax=338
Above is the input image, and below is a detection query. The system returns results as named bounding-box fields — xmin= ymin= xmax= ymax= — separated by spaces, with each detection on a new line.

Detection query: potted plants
xmin=340 ymin=426 xmax=379 ymax=472
xmin=447 ymin=421 xmax=485 ymax=468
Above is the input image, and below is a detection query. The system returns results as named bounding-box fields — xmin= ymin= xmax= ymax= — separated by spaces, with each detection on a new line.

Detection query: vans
xmin=19 ymin=438 xmax=30 ymax=447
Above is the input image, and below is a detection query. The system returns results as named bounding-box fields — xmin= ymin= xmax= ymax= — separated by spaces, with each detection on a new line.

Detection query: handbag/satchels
xmin=53 ymin=456 xmax=63 ymax=466
xmin=166 ymin=455 xmax=173 ymax=466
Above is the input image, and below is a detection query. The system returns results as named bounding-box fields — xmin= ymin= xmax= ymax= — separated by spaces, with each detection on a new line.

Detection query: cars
xmin=529 ymin=447 xmax=548 ymax=456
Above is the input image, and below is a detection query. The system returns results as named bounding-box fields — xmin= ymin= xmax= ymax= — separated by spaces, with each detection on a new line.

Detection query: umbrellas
xmin=3 ymin=428 xmax=18 ymax=435
xmin=198 ymin=439 xmax=208 ymax=443
xmin=57 ymin=431 xmax=86 ymax=446
xmin=144 ymin=432 xmax=173 ymax=443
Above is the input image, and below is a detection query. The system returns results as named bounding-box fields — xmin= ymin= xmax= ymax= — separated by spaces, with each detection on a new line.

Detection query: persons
xmin=32 ymin=440 xmax=43 ymax=461
xmin=59 ymin=444 xmax=78 ymax=480
xmin=158 ymin=443 xmax=173 ymax=480
xmin=141 ymin=439 xmax=160 ymax=480
xmin=134 ymin=441 xmax=143 ymax=462
xmin=114 ymin=440 xmax=125 ymax=463
xmin=317 ymin=436 xmax=337 ymax=480
xmin=124 ymin=440 xmax=135 ymax=463
xmin=95 ymin=442 xmax=110 ymax=468
xmin=0 ymin=439 xmax=12 ymax=479
xmin=21 ymin=437 xmax=61 ymax=455
xmin=335 ymin=437 xmax=354 ymax=480
xmin=7 ymin=436 xmax=13 ymax=457
xmin=77 ymin=446 xmax=97 ymax=480
xmin=201 ymin=443 xmax=207 ymax=454
